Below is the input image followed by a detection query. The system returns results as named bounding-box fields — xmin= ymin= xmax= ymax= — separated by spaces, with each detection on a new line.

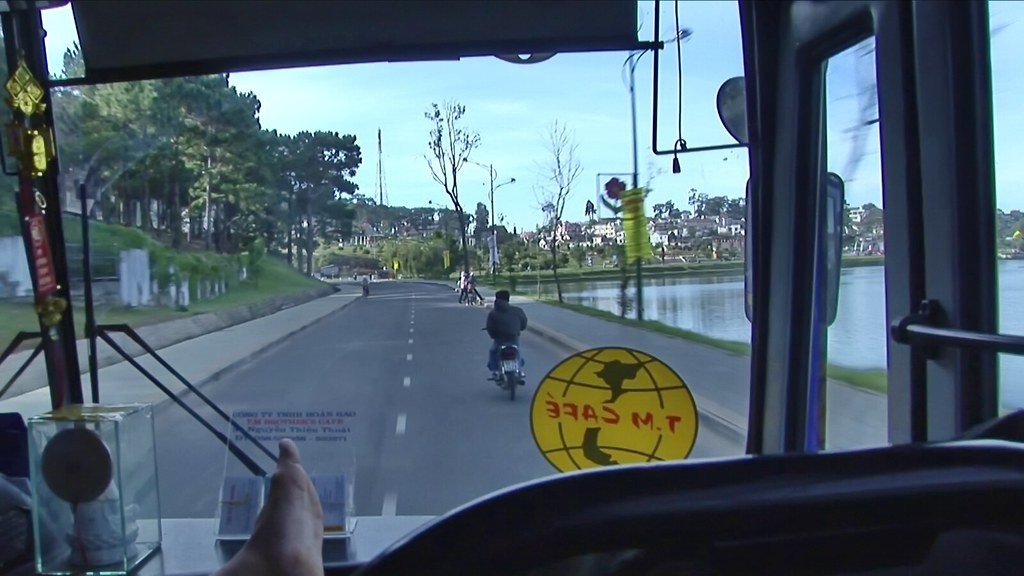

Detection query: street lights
xmin=487 ymin=164 xmax=515 ymax=271
xmin=624 ymin=27 xmax=695 ymax=322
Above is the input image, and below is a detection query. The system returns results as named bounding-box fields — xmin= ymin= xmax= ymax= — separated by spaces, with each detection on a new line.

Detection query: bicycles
xmin=468 ymin=290 xmax=478 ymax=307
xmin=360 ymin=283 xmax=371 ymax=298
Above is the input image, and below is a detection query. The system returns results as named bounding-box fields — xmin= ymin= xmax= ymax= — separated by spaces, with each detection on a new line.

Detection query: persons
xmin=456 ymin=270 xmax=484 ymax=305
xmin=208 ymin=436 xmax=326 ymax=576
xmin=486 ymin=290 xmax=528 ymax=380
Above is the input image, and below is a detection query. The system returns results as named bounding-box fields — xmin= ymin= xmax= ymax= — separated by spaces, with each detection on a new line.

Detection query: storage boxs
xmin=28 ymin=402 xmax=163 ymax=575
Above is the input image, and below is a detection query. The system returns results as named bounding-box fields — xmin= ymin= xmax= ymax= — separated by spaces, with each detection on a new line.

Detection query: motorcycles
xmin=478 ymin=326 xmax=533 ymax=401
